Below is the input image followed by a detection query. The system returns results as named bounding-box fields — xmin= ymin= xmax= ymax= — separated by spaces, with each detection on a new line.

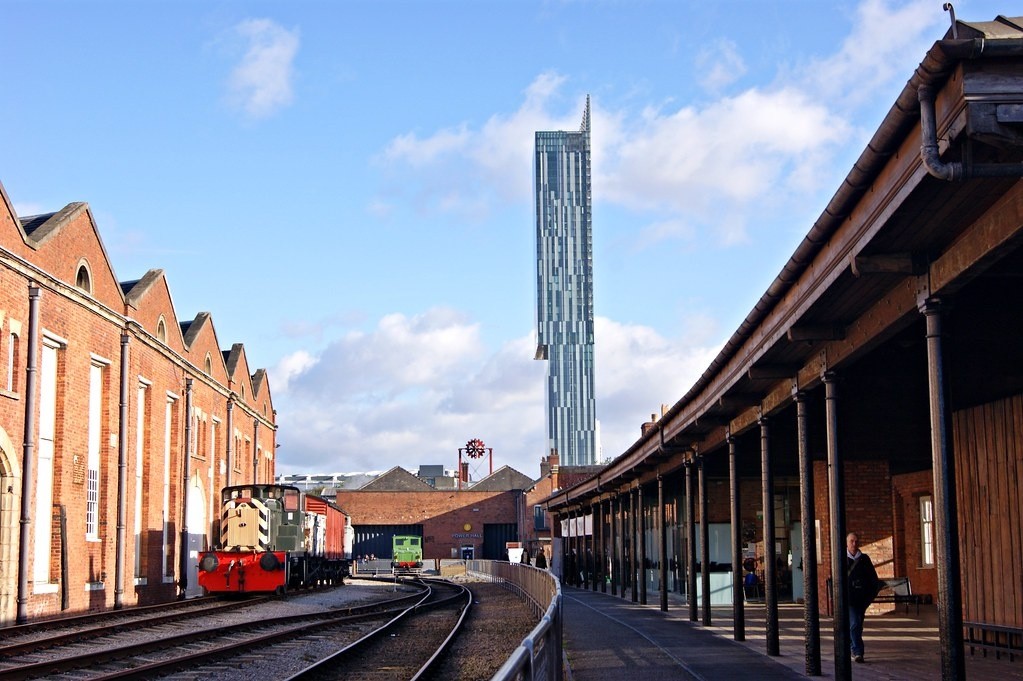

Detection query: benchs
xmin=826 ymin=576 xmax=922 ymax=619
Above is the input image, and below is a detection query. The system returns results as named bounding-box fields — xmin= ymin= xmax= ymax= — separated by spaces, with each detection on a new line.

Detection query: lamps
xmin=473 ymin=508 xmax=480 ymax=512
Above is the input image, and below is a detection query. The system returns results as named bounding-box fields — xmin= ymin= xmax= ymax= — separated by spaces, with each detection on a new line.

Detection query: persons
xmin=502 ymin=549 xmax=510 ymax=561
xmin=534 ymin=547 xmax=549 ymax=568
xmin=520 ymin=548 xmax=531 ymax=566
xmin=465 ymin=552 xmax=473 ymax=560
xmin=845 ymin=532 xmax=879 ymax=662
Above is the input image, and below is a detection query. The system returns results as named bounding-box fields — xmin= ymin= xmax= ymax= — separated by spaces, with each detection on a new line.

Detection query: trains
xmin=193 ymin=483 xmax=355 ymax=601
xmin=390 ymin=534 xmax=423 ymax=576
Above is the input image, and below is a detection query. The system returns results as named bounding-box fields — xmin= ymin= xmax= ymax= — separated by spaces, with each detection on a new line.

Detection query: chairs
xmin=742 ymin=574 xmax=762 ymax=603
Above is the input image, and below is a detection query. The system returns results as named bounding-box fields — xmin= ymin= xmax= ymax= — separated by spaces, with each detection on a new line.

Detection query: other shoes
xmin=851 ymin=654 xmax=865 ymax=664
